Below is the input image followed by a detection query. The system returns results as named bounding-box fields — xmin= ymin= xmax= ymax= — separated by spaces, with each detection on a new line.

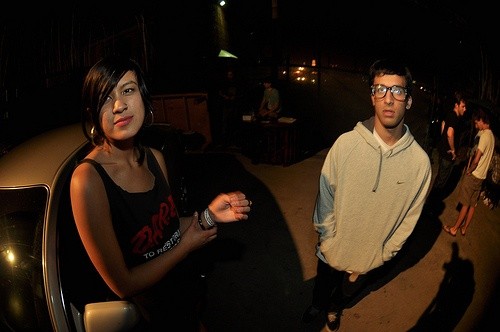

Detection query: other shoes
xmin=442 ymin=224 xmax=465 ymax=236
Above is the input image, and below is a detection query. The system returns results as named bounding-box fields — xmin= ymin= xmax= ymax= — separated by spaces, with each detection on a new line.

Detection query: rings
xmin=249 ymin=200 xmax=253 ymax=206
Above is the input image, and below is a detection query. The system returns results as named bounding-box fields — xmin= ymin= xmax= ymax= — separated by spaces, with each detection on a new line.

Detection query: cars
xmin=0 ymin=120 xmax=190 ymax=332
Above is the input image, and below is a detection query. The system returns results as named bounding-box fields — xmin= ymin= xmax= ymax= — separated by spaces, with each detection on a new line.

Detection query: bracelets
xmin=198 ymin=213 xmax=207 ymax=230
xmin=204 ymin=208 xmax=215 ymax=227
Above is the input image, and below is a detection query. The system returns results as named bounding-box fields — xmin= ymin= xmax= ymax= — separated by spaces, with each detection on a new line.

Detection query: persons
xmin=69 ymin=51 xmax=253 ymax=332
xmin=257 ymin=78 xmax=282 ymax=119
xmin=302 ymin=58 xmax=432 ymax=332
xmin=441 ymin=111 xmax=500 ymax=236
xmin=433 ymin=91 xmax=466 ymax=195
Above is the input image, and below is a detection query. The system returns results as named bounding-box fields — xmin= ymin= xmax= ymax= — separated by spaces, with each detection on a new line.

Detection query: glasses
xmin=369 ymin=85 xmax=410 ymax=101
